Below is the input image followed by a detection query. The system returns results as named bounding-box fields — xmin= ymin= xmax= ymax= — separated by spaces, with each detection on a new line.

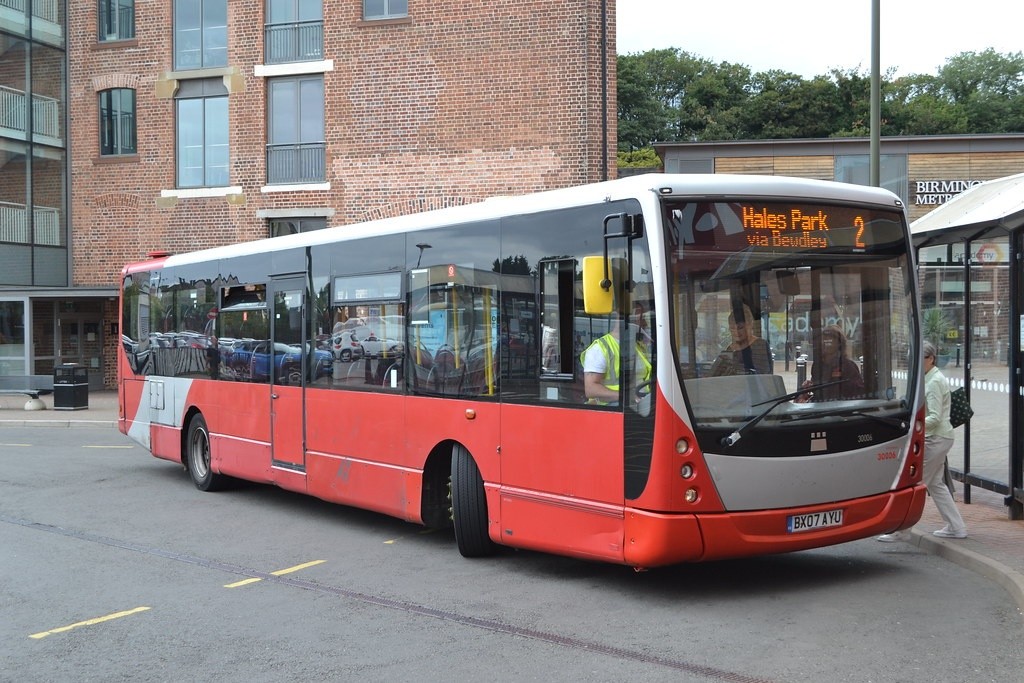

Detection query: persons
xmin=806 ymin=322 xmax=864 ymax=401
xmin=579 ymin=300 xmax=651 ymax=412
xmin=878 ymin=340 xmax=966 ymax=541
xmin=207 ymin=336 xmax=224 ymax=381
xmin=714 ymin=302 xmax=774 ymax=375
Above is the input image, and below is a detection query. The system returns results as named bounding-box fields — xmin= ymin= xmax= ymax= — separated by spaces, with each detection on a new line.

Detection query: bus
xmin=115 ymin=170 xmax=926 ymax=573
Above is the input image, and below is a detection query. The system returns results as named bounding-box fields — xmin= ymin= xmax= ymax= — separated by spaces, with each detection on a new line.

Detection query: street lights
xmin=414 ymin=243 xmax=434 ymax=270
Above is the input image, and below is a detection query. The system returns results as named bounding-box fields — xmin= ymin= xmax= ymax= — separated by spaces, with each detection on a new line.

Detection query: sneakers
xmin=878 ymin=528 xmax=912 ymax=542
xmin=933 ymin=528 xmax=967 ymax=539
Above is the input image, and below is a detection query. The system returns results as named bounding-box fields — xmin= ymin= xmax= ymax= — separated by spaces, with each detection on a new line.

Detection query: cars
xmin=123 ymin=315 xmax=559 ymax=400
xmin=785 ymin=293 xmax=853 ymax=351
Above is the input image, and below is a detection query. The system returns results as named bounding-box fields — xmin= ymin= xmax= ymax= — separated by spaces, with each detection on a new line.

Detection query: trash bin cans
xmin=53 ymin=363 xmax=88 ymax=410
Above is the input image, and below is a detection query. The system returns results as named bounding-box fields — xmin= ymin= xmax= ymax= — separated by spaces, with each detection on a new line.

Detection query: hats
xmin=923 ymin=341 xmax=936 ymax=358
xmin=823 ymin=324 xmax=847 ymax=344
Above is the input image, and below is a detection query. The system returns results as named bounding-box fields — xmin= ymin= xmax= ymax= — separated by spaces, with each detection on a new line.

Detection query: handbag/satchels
xmin=949 ymin=387 xmax=974 ymax=429
